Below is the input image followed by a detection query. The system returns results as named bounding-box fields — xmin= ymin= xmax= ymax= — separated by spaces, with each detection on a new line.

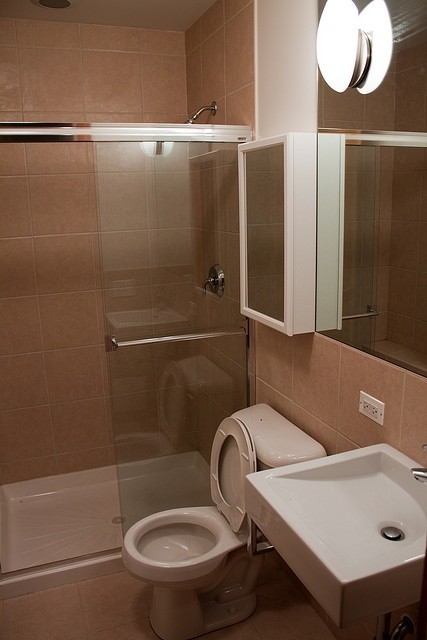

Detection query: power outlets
xmin=357 ymin=389 xmax=384 ymax=426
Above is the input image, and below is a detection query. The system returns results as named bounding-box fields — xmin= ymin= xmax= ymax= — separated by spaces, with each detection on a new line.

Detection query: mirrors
xmin=314 ymin=132 xmax=424 ymax=375
xmin=316 ymin=0 xmax=426 ymax=133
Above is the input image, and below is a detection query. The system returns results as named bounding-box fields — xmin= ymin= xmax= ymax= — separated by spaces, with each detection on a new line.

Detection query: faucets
xmin=411 ymin=444 xmax=427 ymax=482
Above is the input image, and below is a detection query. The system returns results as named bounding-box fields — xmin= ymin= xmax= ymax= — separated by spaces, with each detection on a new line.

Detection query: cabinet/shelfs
xmin=317 ymin=133 xmax=343 ymax=333
xmin=235 ymin=132 xmax=315 ymax=336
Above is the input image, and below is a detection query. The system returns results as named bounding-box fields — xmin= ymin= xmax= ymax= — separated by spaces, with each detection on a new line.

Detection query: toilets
xmin=122 ymin=401 xmax=327 ymax=640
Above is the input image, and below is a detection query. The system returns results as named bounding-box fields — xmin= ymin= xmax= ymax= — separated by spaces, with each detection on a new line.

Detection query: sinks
xmin=246 ymin=442 xmax=427 ymax=627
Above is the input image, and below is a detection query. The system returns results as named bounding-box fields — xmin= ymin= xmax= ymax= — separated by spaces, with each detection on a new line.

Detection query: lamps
xmin=313 ymin=0 xmax=360 ymax=94
xmin=358 ymin=0 xmax=394 ymax=95
